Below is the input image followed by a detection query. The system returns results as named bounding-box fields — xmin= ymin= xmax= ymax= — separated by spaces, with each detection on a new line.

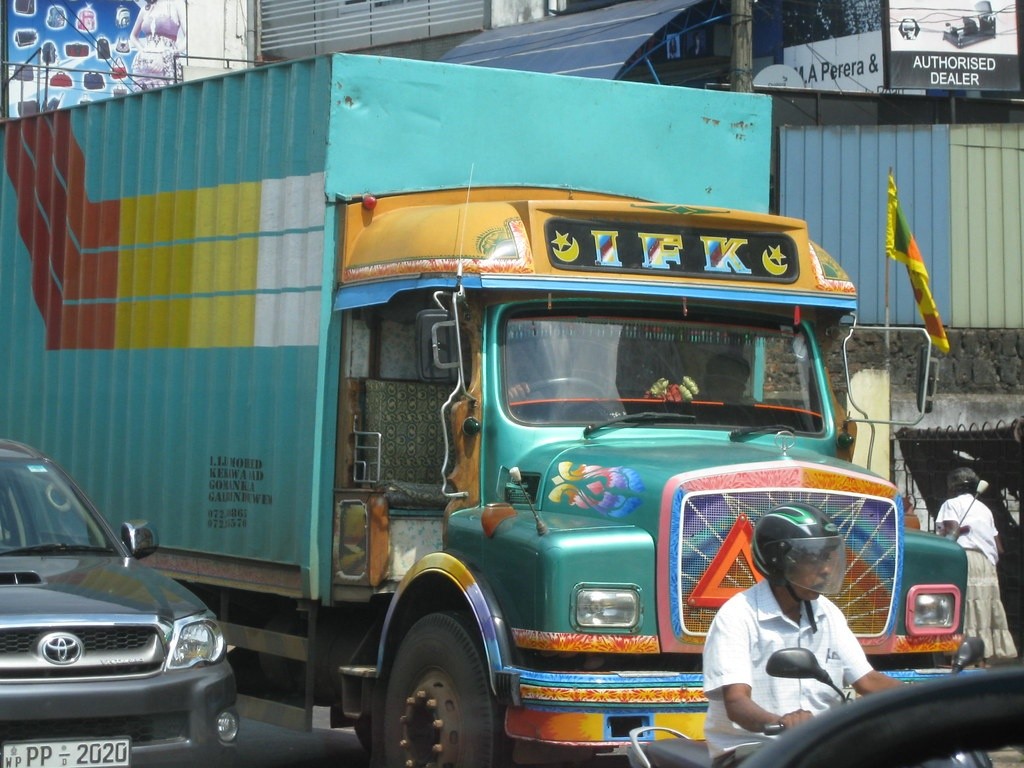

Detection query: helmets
xmin=751 ymin=502 xmax=842 ymax=587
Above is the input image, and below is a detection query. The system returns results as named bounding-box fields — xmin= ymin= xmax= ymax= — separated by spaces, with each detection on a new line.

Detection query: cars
xmin=0 ymin=439 xmax=240 ymax=766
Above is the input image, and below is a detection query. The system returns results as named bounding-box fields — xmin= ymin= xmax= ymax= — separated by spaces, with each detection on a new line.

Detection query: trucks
xmin=0 ymin=51 xmax=991 ymax=766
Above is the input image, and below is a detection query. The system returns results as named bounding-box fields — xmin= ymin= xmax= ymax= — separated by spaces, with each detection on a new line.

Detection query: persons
xmin=507 ymin=331 xmax=596 ymax=400
xmin=934 ymin=468 xmax=1017 ymax=668
xmin=702 ymin=503 xmax=915 ymax=768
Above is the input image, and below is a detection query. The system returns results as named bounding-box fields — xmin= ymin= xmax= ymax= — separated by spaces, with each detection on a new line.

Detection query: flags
xmin=884 ymin=176 xmax=950 ymax=354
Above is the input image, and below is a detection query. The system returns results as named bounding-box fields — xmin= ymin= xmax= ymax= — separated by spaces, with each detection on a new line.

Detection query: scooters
xmin=626 ymin=635 xmax=990 ymax=766
